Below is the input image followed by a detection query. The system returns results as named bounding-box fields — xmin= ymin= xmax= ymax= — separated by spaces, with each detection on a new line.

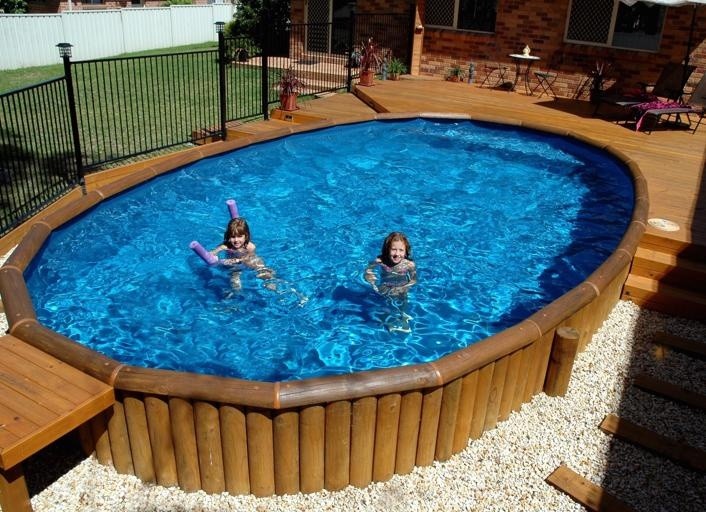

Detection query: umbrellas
xmin=620 ymin=0 xmax=705 ymax=105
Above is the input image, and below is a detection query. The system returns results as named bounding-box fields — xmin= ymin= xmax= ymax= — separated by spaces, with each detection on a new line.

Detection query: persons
xmin=365 ymin=228 xmax=418 ymax=301
xmin=208 ymin=215 xmax=279 ymax=292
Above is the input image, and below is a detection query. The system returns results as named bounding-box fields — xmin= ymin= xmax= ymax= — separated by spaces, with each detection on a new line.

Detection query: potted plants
xmin=269 ymin=64 xmax=307 ymax=112
xmin=347 ymin=36 xmax=408 ymax=87
xmin=449 ymin=66 xmax=462 ymax=83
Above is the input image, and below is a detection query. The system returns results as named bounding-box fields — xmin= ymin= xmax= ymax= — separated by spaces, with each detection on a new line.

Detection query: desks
xmin=507 ymin=53 xmax=540 ymax=95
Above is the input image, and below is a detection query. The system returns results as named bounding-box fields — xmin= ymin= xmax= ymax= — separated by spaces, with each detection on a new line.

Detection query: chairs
xmin=480 ymin=63 xmax=561 ymax=98
xmin=591 ymin=62 xmax=705 ymax=136
